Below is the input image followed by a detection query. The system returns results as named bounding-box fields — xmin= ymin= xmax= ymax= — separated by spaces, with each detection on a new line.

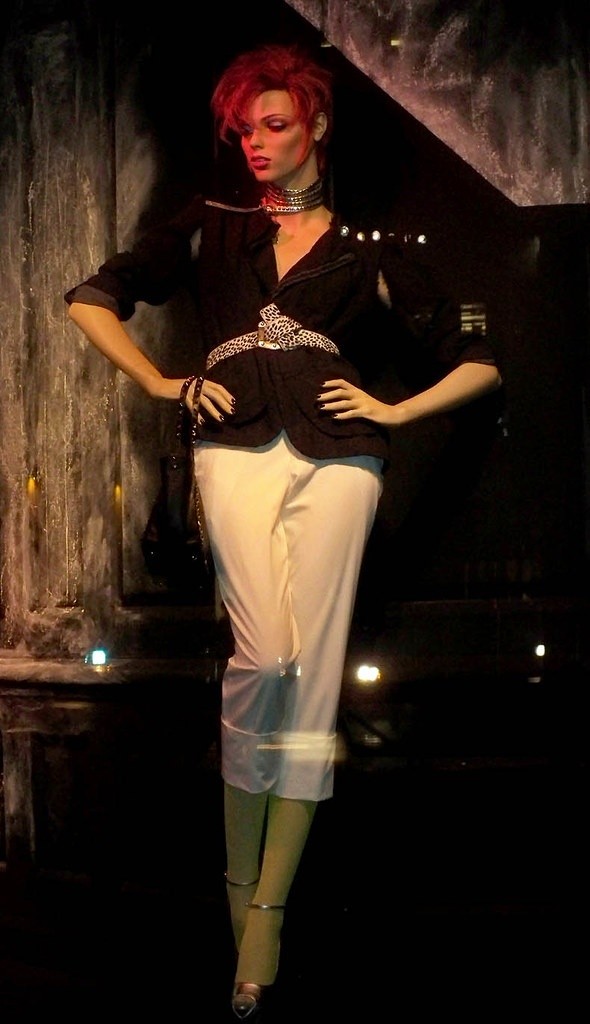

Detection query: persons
xmin=65 ymin=50 xmax=501 ymax=1021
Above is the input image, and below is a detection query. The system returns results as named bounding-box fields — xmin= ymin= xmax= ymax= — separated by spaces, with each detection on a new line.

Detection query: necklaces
xmin=266 ymin=181 xmax=323 ymax=214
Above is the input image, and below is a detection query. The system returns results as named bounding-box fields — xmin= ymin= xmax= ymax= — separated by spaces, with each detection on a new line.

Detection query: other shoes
xmin=232 ymin=900 xmax=286 ymax=1024
xmin=224 ymin=872 xmax=261 ymax=958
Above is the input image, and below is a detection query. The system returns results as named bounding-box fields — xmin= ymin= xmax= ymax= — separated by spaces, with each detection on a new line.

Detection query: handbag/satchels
xmin=141 ymin=372 xmax=215 ymax=598
